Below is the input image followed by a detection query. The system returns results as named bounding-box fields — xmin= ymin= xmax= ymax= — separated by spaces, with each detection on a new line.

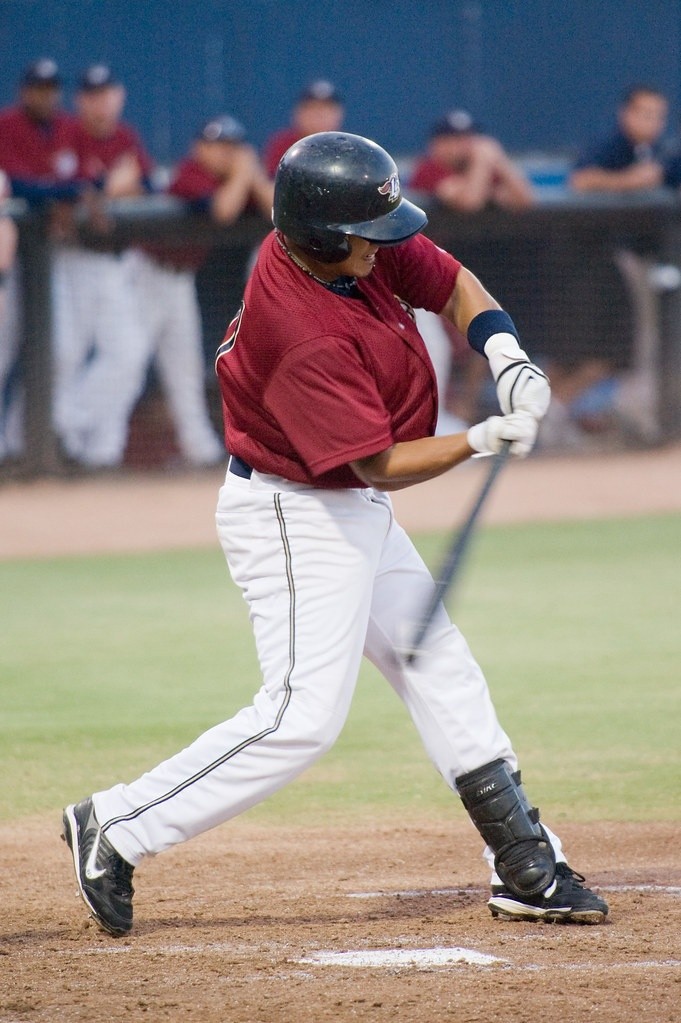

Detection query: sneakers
xmin=487 ymin=866 xmax=609 ymax=924
xmin=63 ymin=797 xmax=136 ymax=939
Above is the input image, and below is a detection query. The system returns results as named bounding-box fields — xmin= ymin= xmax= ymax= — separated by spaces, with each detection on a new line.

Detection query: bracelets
xmin=466 ymin=309 xmax=521 ymax=361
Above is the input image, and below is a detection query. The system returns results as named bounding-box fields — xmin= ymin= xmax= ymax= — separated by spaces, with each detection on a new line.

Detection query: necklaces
xmin=274 ymin=227 xmax=356 ymax=289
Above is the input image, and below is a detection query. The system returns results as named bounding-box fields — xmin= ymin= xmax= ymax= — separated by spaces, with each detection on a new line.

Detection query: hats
xmin=432 ymin=107 xmax=482 ymax=134
xmin=21 ymin=55 xmax=66 ymax=90
xmin=204 ymin=115 xmax=248 ymax=145
xmin=300 ymin=79 xmax=345 ymax=106
xmin=78 ymin=64 xmax=121 ymax=93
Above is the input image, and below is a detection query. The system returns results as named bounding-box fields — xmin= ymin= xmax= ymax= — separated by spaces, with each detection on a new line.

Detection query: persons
xmin=62 ymin=131 xmax=609 ymax=938
xmin=408 ymin=111 xmax=534 ymax=213
xmin=0 ymin=70 xmax=281 ymax=481
xmin=262 ymin=82 xmax=344 ymax=184
xmin=570 ymin=84 xmax=681 ymax=192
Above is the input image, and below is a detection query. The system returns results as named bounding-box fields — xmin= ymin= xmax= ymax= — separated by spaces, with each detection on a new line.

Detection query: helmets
xmin=272 ymin=132 xmax=429 ymax=264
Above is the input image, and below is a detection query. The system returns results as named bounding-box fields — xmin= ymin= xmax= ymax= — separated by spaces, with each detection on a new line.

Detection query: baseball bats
xmin=395 ymin=434 xmax=511 ymax=670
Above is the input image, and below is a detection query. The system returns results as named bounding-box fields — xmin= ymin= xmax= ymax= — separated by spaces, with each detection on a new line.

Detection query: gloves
xmin=469 ymin=350 xmax=554 ymax=460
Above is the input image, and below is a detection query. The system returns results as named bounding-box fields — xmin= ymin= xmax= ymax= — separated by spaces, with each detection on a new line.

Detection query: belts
xmin=229 ymin=457 xmax=254 ymax=480
